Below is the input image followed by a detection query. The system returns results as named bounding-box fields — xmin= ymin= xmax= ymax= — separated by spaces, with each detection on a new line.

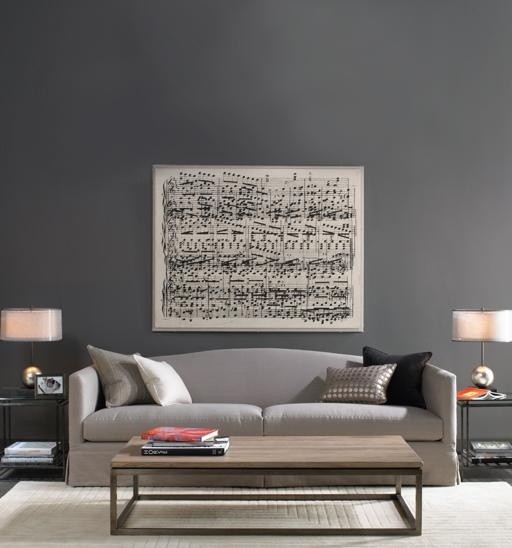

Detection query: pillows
xmin=320 ymin=363 xmax=397 ymax=404
xmin=84 ymin=342 xmax=153 ymax=409
xmin=358 ymin=346 xmax=435 ymax=405
xmin=133 ymin=352 xmax=193 ymax=405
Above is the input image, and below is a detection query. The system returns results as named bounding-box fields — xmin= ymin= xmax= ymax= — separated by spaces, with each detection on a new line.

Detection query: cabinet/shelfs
xmin=458 ymin=398 xmax=512 ymax=481
xmin=0 ymin=390 xmax=68 ymax=482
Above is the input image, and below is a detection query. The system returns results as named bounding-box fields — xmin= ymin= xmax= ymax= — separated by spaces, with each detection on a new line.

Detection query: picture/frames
xmin=152 ymin=164 xmax=368 ymax=332
xmin=33 ymin=374 xmax=65 ymax=399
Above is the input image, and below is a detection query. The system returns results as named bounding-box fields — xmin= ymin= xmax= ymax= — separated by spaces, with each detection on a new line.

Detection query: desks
xmin=106 ymin=434 xmax=429 ymax=542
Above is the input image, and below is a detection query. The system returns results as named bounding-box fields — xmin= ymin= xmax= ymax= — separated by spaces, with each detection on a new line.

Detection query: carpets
xmin=0 ymin=479 xmax=512 ymax=548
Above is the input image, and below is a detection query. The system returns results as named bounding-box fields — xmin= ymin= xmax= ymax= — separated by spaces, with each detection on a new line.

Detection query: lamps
xmin=450 ymin=306 xmax=509 ymax=408
xmin=0 ymin=309 xmax=60 ymax=387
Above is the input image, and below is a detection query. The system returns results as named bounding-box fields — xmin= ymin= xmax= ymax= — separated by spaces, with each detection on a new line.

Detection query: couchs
xmin=67 ymin=347 xmax=463 ymax=491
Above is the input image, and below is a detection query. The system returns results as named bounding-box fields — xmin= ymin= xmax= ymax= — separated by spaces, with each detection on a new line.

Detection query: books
xmin=457 ymin=386 xmax=508 ymax=402
xmin=460 ymin=439 xmax=512 ymax=464
xmin=1 ymin=438 xmax=62 ymax=464
xmin=139 ymin=425 xmax=231 ymax=456
